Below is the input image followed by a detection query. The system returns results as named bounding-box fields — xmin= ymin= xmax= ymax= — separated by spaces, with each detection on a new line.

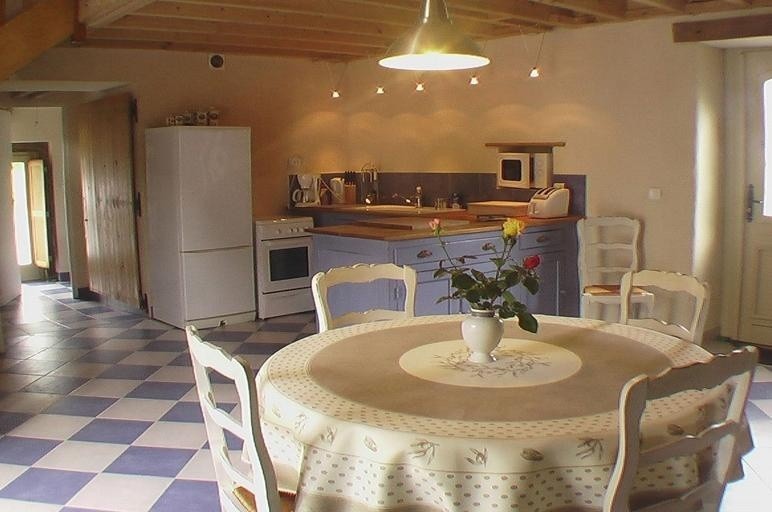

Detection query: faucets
xmin=392 ymin=193 xmax=422 ymax=208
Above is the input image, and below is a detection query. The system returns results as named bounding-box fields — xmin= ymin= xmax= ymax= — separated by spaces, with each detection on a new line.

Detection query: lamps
xmin=325 ymin=62 xmax=349 ymax=98
xmin=470 ymin=39 xmax=487 ymax=84
xmin=370 ymin=57 xmax=392 ymax=94
xmin=518 ymin=24 xmax=545 ymax=78
xmin=415 ymin=71 xmax=425 ymax=92
xmin=377 ymin=1 xmax=492 ymax=71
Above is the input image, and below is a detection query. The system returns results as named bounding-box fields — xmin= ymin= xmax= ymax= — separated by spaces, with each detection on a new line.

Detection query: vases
xmin=462 ymin=308 xmax=504 ymax=363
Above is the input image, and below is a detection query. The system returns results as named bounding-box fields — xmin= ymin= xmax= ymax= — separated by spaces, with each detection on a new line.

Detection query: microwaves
xmin=496 ymin=152 xmax=553 ymax=189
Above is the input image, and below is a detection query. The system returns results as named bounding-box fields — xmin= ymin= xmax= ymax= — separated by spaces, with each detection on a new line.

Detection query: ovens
xmin=254 ymin=215 xmax=315 ymax=320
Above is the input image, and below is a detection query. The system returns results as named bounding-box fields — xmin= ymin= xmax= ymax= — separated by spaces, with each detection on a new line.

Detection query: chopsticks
xmin=344 ymin=170 xmax=356 ymax=186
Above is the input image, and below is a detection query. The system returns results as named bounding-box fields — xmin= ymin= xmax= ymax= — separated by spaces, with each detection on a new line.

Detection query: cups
xmin=432 ymin=199 xmax=448 ymax=210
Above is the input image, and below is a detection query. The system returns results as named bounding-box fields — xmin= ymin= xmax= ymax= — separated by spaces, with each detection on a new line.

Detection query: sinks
xmin=356 ymin=205 xmax=416 ymax=208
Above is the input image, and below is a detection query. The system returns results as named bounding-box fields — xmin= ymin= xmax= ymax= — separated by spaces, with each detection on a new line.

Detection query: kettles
xmin=330 ymin=179 xmax=346 ymax=205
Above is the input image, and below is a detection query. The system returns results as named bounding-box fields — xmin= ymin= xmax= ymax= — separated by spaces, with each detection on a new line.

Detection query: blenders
xmin=295 ymin=174 xmax=322 ymax=209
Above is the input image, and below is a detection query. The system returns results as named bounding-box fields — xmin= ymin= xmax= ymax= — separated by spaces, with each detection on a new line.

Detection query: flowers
xmin=418 ymin=218 xmax=540 ymax=333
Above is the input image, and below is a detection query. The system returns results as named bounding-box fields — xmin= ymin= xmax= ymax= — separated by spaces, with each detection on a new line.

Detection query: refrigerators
xmin=144 ymin=125 xmax=257 ymax=331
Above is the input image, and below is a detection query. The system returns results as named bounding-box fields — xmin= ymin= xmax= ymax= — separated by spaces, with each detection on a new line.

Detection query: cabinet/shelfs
xmin=313 ymin=220 xmax=580 ymax=330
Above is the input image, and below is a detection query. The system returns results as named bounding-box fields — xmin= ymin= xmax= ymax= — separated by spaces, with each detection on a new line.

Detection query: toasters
xmin=526 ymin=186 xmax=570 ymax=219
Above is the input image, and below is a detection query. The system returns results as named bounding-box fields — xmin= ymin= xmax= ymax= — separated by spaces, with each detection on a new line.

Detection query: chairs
xmin=619 ymin=270 xmax=711 ymax=346
xmin=577 ymin=216 xmax=654 ymax=318
xmin=603 ymin=345 xmax=758 ymax=512
xmin=312 ymin=263 xmax=418 ymax=332
xmin=185 ymin=325 xmax=296 ymax=511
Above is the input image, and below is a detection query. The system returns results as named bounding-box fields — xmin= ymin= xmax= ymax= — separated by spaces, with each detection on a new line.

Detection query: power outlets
xmin=649 ymin=189 xmax=661 ymax=199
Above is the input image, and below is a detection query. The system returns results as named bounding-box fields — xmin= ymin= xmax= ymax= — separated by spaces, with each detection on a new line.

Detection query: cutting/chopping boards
xmin=351 ymin=216 xmax=470 ymax=229
